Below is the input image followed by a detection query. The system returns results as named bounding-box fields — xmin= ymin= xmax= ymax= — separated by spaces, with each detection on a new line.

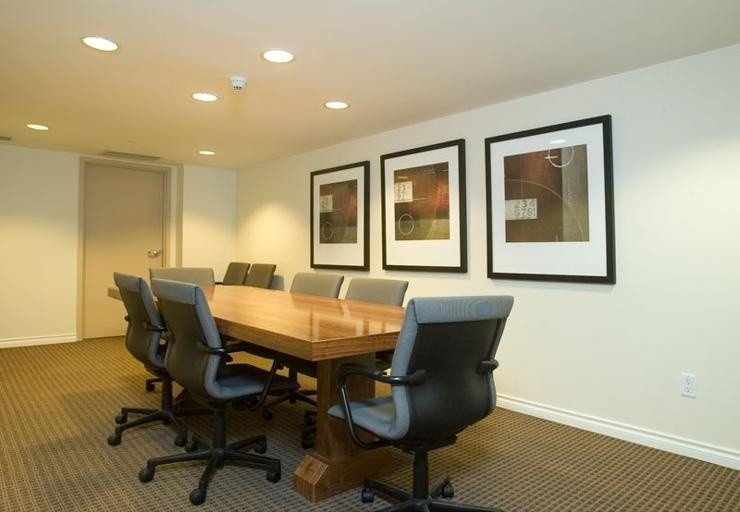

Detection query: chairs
xmin=139 ymin=277 xmax=301 ymax=505
xmin=302 ymin=278 xmax=409 ymax=449
xmin=261 ymin=272 xmax=344 ymax=418
xmin=148 ymin=267 xmax=214 ymax=284
xmin=222 ymin=262 xmax=250 ymax=285
xmin=107 ymin=271 xmax=214 ymax=454
xmin=325 ymin=295 xmax=515 ymax=512
xmin=244 ymin=263 xmax=276 ymax=289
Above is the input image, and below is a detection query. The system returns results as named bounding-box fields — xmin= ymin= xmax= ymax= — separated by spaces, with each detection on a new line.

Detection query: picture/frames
xmin=380 ymin=137 xmax=468 ymax=274
xmin=484 ymin=114 xmax=616 ymax=285
xmin=308 ymin=160 xmax=370 ymax=272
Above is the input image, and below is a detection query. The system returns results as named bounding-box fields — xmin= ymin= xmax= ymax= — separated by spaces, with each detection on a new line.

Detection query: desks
xmin=107 ymin=283 xmax=406 ymax=504
